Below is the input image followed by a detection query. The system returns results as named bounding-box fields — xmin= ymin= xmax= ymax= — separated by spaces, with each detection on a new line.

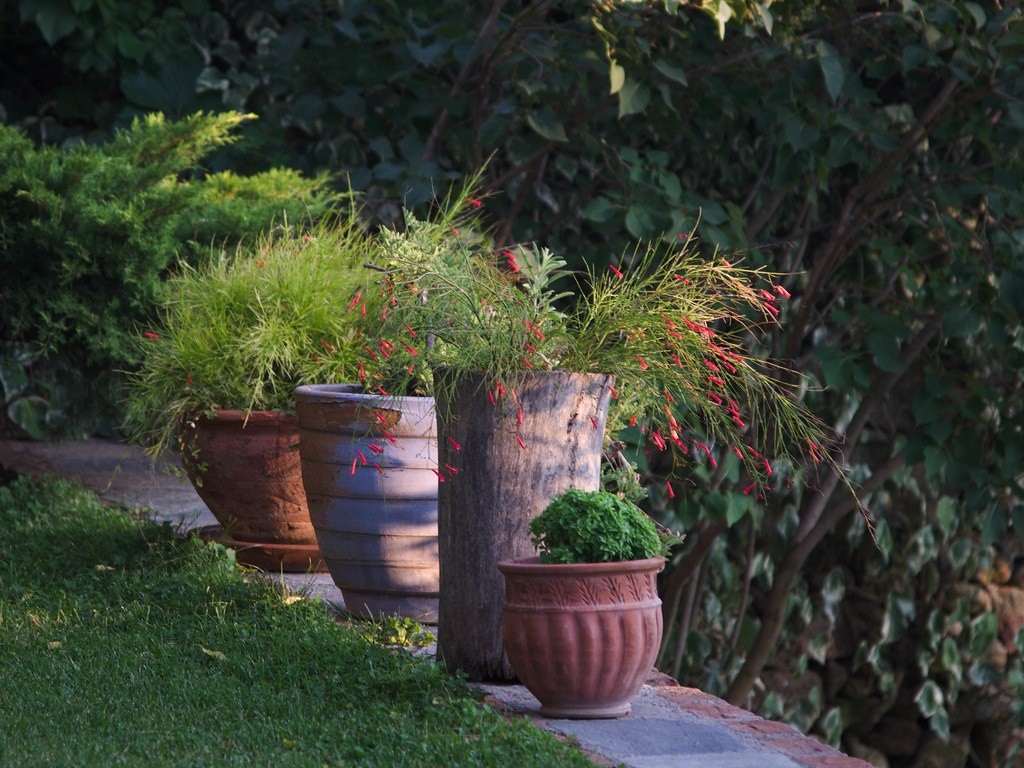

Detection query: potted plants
xmin=497 ymin=488 xmax=665 ymax=720
xmin=121 ymin=206 xmax=369 ymax=575
xmin=293 ymin=264 xmax=441 ymax=626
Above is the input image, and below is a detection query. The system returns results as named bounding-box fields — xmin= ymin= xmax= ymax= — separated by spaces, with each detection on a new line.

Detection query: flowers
xmin=311 ymin=183 xmax=882 ymax=557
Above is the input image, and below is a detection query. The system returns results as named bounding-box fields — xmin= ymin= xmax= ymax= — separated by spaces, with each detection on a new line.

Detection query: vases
xmin=429 ymin=363 xmax=615 ymax=684
